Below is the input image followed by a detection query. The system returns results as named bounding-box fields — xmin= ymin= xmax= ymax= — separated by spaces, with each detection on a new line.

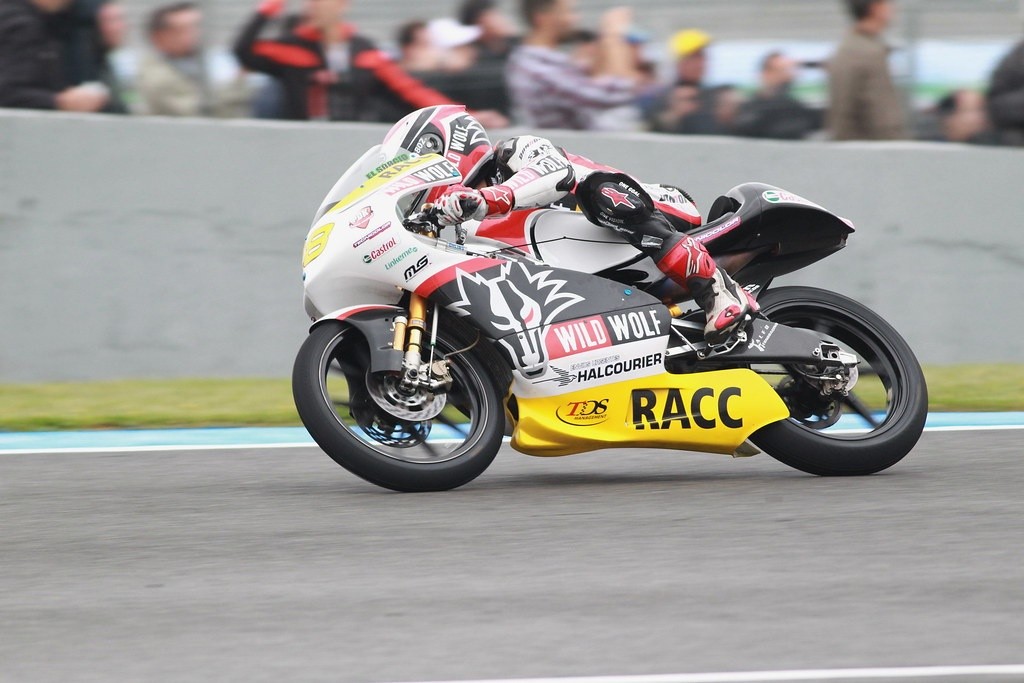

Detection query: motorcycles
xmin=291 ymin=144 xmax=930 ymax=493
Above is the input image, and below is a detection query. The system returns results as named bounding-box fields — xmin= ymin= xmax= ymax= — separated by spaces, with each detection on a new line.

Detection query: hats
xmin=428 ymin=18 xmax=482 ymax=49
xmin=673 ymin=29 xmax=708 ymax=56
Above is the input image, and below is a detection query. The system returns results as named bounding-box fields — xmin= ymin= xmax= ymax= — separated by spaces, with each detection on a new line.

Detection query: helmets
xmin=382 ymin=104 xmax=494 ymax=220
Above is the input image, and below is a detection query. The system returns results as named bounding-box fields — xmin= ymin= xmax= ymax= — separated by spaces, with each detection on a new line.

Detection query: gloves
xmin=434 ymin=184 xmax=486 ymax=226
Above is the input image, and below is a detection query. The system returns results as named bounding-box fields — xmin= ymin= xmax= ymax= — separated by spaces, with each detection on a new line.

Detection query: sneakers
xmin=704 ymin=265 xmax=760 ymax=344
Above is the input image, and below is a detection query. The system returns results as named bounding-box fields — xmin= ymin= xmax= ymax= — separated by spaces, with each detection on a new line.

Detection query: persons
xmin=0 ymin=0 xmax=1024 ymax=146
xmin=383 ymin=105 xmax=762 ymax=343
